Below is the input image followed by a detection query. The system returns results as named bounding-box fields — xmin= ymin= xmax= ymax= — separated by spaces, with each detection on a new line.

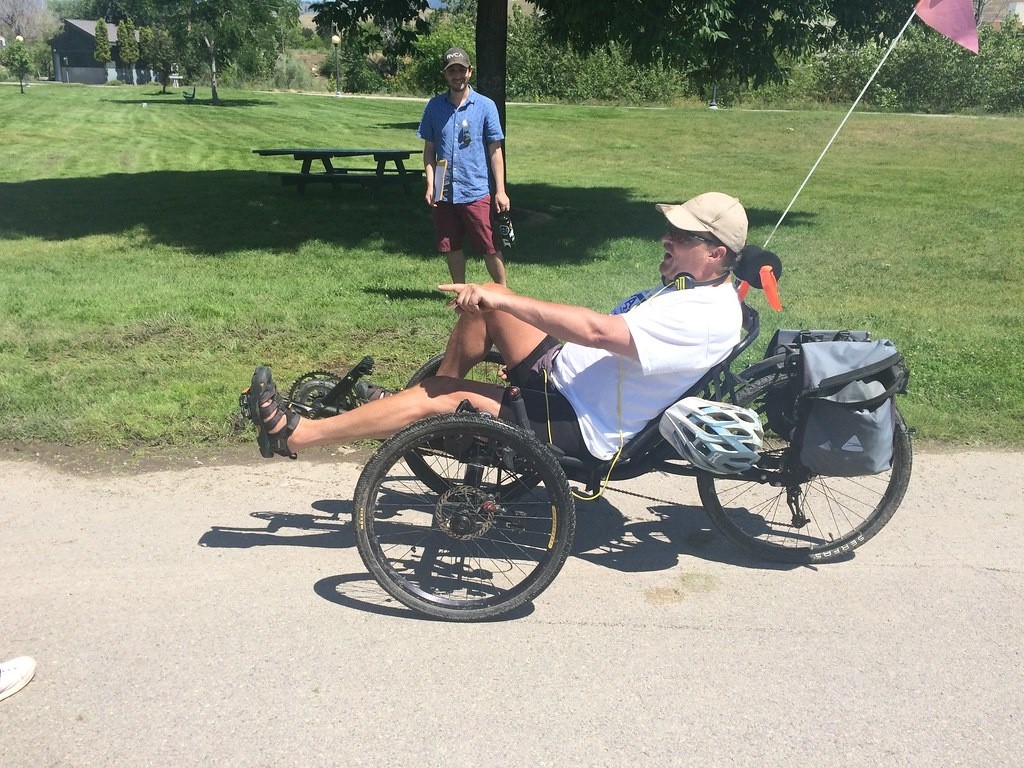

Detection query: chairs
xmin=560 ymin=301 xmax=760 ymax=476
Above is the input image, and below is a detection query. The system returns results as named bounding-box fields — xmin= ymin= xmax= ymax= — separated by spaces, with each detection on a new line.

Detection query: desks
xmin=252 ymin=148 xmax=423 ymax=199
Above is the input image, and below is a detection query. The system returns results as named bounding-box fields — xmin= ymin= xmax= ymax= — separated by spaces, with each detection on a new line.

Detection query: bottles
xmin=498 ymin=211 xmax=516 ymax=248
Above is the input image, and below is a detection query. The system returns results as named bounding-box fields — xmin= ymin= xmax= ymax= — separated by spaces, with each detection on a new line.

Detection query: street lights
xmin=332 ymin=35 xmax=342 ymax=95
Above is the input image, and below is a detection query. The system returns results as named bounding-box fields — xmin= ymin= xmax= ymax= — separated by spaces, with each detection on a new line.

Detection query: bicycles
xmin=240 ymin=244 xmax=912 ymax=622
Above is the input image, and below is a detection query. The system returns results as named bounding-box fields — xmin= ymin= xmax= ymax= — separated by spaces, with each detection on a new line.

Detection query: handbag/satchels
xmin=769 ymin=327 xmax=904 ymax=476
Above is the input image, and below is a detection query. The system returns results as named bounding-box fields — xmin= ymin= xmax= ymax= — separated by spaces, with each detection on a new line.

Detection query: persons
xmin=249 ymin=192 xmax=748 ymax=461
xmin=416 ymin=48 xmax=511 ymax=307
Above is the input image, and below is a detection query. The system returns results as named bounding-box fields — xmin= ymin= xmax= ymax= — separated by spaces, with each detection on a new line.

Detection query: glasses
xmin=664 ymin=224 xmax=714 ymax=246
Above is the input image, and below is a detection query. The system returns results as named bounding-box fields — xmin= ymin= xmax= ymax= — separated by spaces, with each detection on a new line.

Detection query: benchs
xmin=330 ymin=167 xmax=425 ymax=174
xmin=269 ymin=171 xmax=384 ymax=183
xmin=182 ymin=86 xmax=196 ymax=104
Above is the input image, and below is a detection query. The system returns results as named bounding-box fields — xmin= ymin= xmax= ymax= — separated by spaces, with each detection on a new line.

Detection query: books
xmin=433 ymin=160 xmax=447 ymax=202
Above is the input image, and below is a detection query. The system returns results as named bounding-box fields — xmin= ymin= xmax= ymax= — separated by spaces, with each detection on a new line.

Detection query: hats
xmin=441 ymin=47 xmax=472 ymax=71
xmin=654 ymin=192 xmax=749 ymax=254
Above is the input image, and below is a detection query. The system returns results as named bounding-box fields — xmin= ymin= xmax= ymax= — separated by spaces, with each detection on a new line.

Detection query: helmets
xmin=658 ymin=396 xmax=764 ymax=475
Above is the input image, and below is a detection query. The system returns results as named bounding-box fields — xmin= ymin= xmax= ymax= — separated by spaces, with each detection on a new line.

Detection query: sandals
xmin=357 ymin=380 xmax=402 ymax=404
xmin=248 ymin=365 xmax=302 ymax=460
